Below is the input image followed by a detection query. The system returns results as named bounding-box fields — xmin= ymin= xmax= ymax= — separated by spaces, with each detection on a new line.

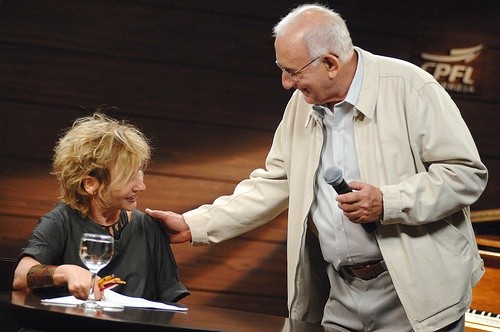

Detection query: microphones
xmin=323 ymin=165 xmax=377 ymax=233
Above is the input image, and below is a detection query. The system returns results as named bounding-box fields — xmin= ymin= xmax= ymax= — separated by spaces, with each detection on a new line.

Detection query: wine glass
xmin=74 ymin=233 xmax=114 ymax=308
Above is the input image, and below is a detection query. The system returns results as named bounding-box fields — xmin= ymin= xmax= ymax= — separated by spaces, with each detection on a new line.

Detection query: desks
xmin=0 ymin=290 xmax=343 ymax=332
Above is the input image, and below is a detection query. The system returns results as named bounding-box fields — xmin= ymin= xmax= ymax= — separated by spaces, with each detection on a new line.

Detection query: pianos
xmin=464 ymin=209 xmax=500 ymax=332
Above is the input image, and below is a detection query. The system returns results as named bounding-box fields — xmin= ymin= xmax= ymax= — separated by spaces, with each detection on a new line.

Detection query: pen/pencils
xmin=97 ymin=274 xmax=127 ymax=293
